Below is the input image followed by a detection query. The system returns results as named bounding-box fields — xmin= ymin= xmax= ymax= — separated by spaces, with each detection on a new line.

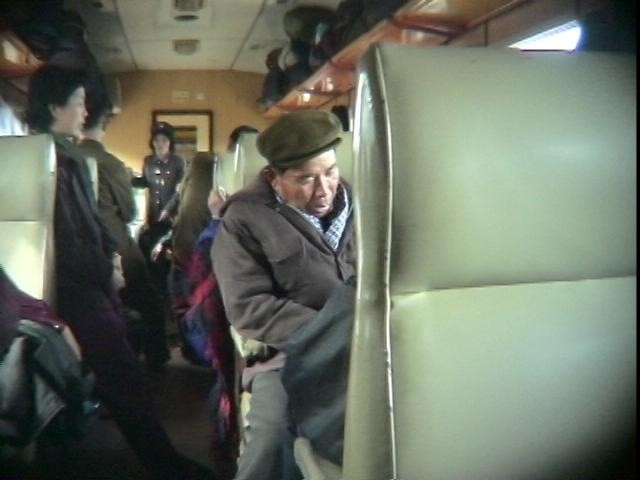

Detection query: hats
xmin=150 ymin=120 xmax=177 ymax=153
xmin=254 ymin=107 xmax=344 ymax=171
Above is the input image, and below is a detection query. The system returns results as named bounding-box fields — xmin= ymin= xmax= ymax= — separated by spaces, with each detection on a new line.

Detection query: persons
xmin=127 ymin=121 xmax=188 ymax=339
xmin=25 ymin=62 xmax=222 ymax=479
xmin=169 ymin=124 xmax=261 ymax=270
xmin=71 ymin=92 xmax=174 ymax=369
xmin=151 ymin=227 xmax=174 ymax=264
xmin=173 ymin=183 xmax=239 ymax=479
xmin=210 ymin=108 xmax=358 ymax=479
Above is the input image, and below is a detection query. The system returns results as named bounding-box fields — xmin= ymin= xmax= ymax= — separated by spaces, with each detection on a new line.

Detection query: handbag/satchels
xmin=1 ymin=317 xmax=103 ymax=480
xmin=255 ymin=0 xmax=402 ymax=106
xmin=1 ymin=0 xmax=113 ymax=107
